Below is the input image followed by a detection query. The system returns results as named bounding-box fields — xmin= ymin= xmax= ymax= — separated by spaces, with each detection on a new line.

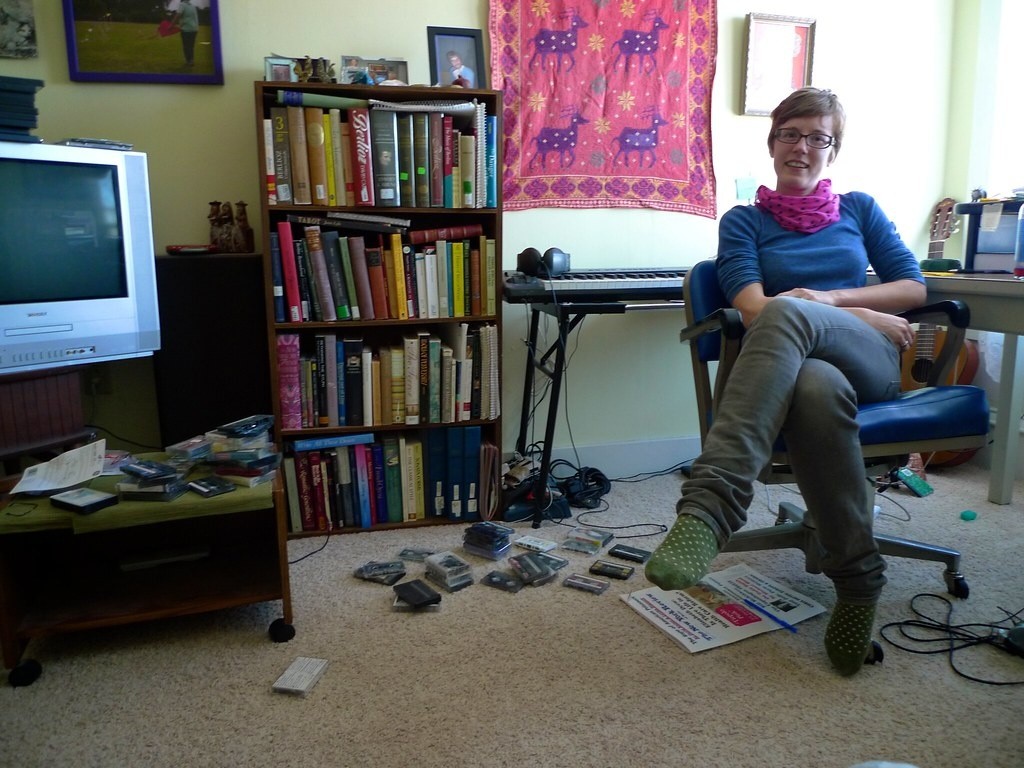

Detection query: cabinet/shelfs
xmin=254 ymin=80 xmax=504 ymax=538
xmin=152 ymin=253 xmax=273 ymax=449
xmin=0 ymin=451 xmax=296 ymax=688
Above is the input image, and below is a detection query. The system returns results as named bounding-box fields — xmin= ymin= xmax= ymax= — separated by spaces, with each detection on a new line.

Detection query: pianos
xmin=501 ymin=266 xmax=694 ymax=529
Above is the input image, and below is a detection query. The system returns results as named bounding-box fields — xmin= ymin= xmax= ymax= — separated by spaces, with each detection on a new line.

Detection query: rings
xmin=902 ymin=340 xmax=909 ymax=348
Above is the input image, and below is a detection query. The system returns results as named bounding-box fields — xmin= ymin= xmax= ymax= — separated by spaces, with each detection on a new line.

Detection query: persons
xmin=168 ymin=0 xmax=199 ymax=67
xmin=645 ymin=87 xmax=927 ymax=677
xmin=446 ymin=52 xmax=475 ymax=88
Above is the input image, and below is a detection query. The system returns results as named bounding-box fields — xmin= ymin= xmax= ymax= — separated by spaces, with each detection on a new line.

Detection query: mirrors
xmin=740 ymin=12 xmax=817 ymax=117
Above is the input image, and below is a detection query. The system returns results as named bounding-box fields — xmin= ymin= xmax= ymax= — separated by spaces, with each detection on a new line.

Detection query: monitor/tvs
xmin=0 ymin=142 xmax=162 ymax=375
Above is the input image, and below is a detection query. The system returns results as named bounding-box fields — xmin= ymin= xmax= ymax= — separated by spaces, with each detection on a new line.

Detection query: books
xmin=263 ymin=89 xmax=501 ymax=533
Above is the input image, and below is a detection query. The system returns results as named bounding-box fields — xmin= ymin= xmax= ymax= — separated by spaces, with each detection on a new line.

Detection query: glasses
xmin=773 ymin=126 xmax=838 ymax=149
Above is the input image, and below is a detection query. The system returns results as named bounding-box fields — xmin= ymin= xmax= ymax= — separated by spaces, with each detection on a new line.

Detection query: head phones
xmin=515 ymin=247 xmax=571 ymax=276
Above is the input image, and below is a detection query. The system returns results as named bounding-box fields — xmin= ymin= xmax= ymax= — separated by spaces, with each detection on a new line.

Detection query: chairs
xmin=679 ymin=261 xmax=991 ymax=666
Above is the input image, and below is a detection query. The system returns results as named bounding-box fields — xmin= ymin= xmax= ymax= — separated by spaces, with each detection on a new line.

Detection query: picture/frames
xmin=62 ymin=0 xmax=224 ymax=86
xmin=265 ymin=57 xmax=299 ymax=82
xmin=427 ymin=26 xmax=486 ymax=89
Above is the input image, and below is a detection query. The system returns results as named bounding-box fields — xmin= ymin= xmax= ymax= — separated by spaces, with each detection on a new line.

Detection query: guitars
xmin=881 ymin=197 xmax=981 ymax=471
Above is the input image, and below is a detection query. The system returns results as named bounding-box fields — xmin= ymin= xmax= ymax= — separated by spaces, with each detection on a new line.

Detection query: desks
xmin=863 ymin=271 xmax=1024 ymax=506
xmin=1 ymin=364 xmax=96 ymax=464
